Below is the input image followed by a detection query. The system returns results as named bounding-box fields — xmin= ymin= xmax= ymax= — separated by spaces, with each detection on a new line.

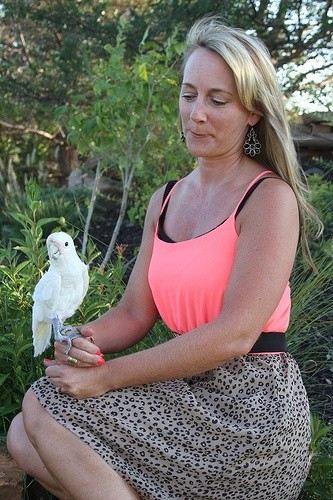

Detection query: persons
xmin=7 ymin=15 xmax=327 ymax=500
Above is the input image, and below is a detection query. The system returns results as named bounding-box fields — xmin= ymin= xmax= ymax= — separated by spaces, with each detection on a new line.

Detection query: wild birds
xmin=31 ymin=231 xmax=86 ymax=358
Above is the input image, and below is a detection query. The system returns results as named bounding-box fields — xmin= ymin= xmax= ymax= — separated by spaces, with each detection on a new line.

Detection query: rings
xmin=55 ymin=385 xmax=64 ymax=395
xmin=66 ymin=355 xmax=78 ymax=366
